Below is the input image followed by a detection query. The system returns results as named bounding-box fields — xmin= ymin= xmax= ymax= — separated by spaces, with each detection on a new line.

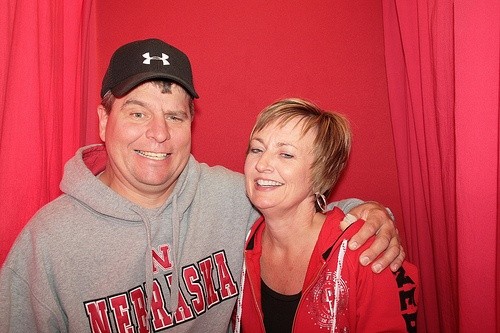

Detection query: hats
xmin=100 ymin=38 xmax=200 ymax=99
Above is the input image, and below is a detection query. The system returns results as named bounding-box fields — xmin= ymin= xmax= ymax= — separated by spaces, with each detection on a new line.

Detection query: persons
xmin=0 ymin=37 xmax=405 ymax=332
xmin=230 ymin=96 xmax=421 ymax=332
